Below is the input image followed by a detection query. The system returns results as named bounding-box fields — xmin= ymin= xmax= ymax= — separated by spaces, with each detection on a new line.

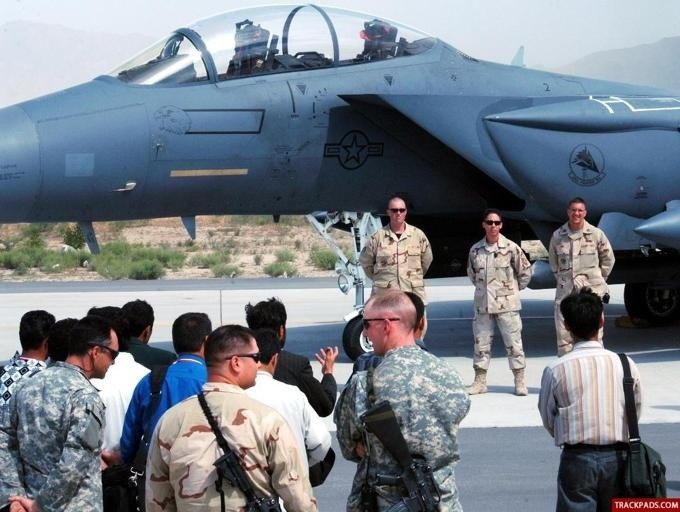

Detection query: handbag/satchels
xmin=618 ymin=353 xmax=667 ymax=498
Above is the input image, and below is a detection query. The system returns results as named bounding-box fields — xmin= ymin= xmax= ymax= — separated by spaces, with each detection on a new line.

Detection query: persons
xmin=221 ymin=26 xmax=269 ymax=84
xmin=249 ymin=328 xmax=331 ymax=468
xmin=100 ymin=312 xmax=219 ymax=512
xmin=549 ymin=198 xmax=617 ymax=361
xmin=351 ymin=25 xmax=392 ymax=69
xmin=145 ymin=325 xmax=319 ymax=512
xmin=331 ymin=289 xmax=473 ymax=511
xmin=1 ymin=314 xmax=107 ymax=511
xmin=120 ymin=299 xmax=178 ymax=390
xmin=540 ymin=289 xmax=645 ymax=511
xmin=240 ymin=295 xmax=338 ymax=416
xmin=461 ymin=209 xmax=532 ymax=396
xmin=76 ymin=307 xmax=150 ymax=453
xmin=2 ymin=310 xmax=65 ymax=512
xmin=9 ymin=321 xmax=98 ymax=452
xmin=359 ymin=197 xmax=434 ymax=347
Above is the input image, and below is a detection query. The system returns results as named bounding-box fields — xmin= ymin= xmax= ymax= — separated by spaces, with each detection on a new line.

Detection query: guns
xmin=359 ymin=404 xmax=442 ymax=512
xmin=211 ymin=451 xmax=283 ymax=512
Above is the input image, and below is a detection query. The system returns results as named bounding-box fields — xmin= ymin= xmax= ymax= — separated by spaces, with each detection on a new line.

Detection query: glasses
xmin=484 ymin=220 xmax=502 ymax=225
xmin=362 ymin=318 xmax=400 ymax=329
xmin=225 ymin=352 xmax=261 ymax=364
xmin=87 ymin=343 xmax=119 ymax=360
xmin=389 ymin=208 xmax=406 ymax=213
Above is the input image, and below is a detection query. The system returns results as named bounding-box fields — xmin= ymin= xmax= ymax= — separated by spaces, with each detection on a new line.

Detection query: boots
xmin=465 ymin=369 xmax=488 ymax=395
xmin=513 ymin=368 xmax=528 ymax=396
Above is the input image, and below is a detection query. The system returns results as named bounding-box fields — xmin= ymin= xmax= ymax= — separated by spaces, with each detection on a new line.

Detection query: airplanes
xmin=1 ymin=3 xmax=679 ymax=361
